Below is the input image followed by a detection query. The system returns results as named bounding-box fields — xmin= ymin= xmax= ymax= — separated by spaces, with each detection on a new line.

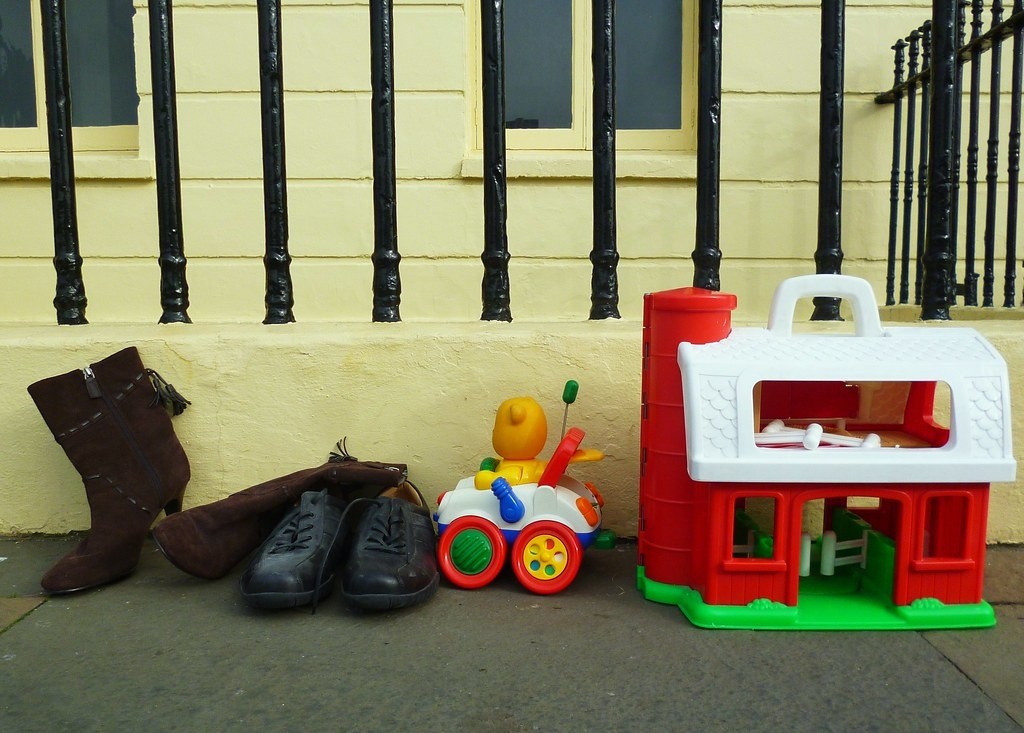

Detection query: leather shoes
xmin=240 ymin=479 xmax=439 ymax=608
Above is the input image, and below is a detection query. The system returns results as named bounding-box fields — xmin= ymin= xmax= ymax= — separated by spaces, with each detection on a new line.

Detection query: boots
xmin=153 ymin=438 xmax=407 ymax=580
xmin=27 ymin=347 xmax=191 ymax=595
